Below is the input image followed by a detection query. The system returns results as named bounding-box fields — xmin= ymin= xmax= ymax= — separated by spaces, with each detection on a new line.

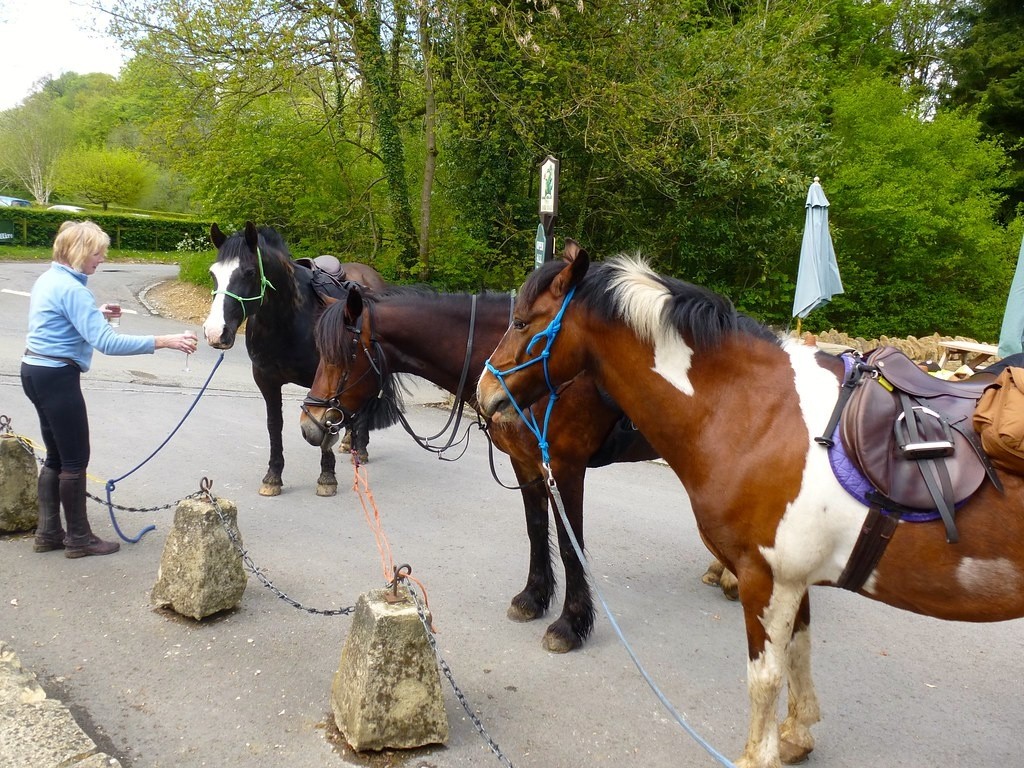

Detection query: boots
xmin=59 ymin=469 xmax=121 ymax=558
xmin=33 ymin=465 xmax=66 ymax=553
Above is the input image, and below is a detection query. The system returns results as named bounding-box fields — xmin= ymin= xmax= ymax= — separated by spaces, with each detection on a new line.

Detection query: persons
xmin=20 ymin=219 xmax=201 ymax=558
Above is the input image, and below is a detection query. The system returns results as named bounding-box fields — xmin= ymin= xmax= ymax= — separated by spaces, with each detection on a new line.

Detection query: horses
xmin=298 ymin=283 xmax=745 ymax=653
xmin=473 ymin=237 xmax=1024 ymax=768
xmin=203 ymin=219 xmax=419 ymax=496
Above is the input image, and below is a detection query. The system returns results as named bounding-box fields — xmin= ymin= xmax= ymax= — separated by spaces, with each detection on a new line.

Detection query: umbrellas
xmin=792 ymin=176 xmax=846 ymax=340
